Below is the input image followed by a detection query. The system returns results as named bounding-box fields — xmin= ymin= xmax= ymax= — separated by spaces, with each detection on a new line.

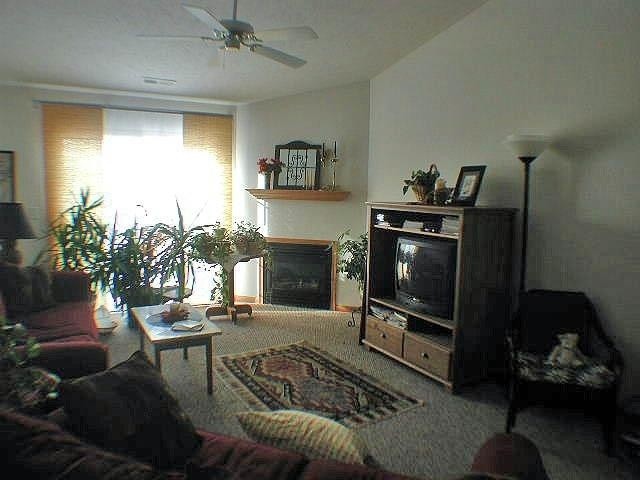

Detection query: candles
xmin=322 ymin=143 xmax=324 ymax=156
xmin=434 ymin=177 xmax=446 ymax=192
xmin=335 ymin=141 xmax=336 ymax=158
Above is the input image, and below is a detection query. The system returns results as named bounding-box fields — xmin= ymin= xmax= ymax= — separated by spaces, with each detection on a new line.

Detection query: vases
xmin=264 ymin=171 xmax=271 ymax=190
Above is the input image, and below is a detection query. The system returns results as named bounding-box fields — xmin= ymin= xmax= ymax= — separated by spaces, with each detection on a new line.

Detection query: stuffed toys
xmin=547 ymin=331 xmax=591 ymax=371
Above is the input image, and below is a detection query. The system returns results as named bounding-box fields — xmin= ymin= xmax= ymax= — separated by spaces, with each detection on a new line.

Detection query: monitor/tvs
xmin=394 ymin=235 xmax=456 ymax=319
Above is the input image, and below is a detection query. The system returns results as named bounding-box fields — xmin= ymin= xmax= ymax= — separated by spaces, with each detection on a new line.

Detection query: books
xmin=172 ymin=320 xmax=206 ymax=333
xmin=369 ymin=301 xmax=409 ymax=331
xmin=401 ymin=212 xmax=461 ymax=236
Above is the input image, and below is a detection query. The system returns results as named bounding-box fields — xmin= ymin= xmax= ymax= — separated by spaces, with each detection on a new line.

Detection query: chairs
xmin=507 ymin=288 xmax=625 ymax=458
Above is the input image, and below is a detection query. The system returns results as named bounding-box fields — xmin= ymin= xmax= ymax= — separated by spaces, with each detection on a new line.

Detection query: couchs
xmin=0 ymin=270 xmax=111 ymax=376
xmin=1 ymin=367 xmax=549 ymax=480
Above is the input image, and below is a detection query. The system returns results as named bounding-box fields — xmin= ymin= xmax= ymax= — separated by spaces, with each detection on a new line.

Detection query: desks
xmin=188 ymin=249 xmax=268 ymax=324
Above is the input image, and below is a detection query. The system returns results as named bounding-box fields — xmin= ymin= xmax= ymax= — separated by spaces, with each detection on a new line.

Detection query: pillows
xmin=236 ymin=407 xmax=371 ymax=465
xmin=1 ymin=364 xmax=64 ymax=419
xmin=0 ymin=261 xmax=57 ymax=317
xmin=56 ymin=348 xmax=206 ymax=472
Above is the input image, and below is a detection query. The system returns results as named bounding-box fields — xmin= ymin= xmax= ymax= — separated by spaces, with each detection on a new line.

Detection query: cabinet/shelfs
xmin=357 ymin=202 xmax=521 ymax=396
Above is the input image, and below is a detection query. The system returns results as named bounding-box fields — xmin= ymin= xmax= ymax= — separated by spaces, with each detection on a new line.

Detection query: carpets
xmin=213 ymin=340 xmax=425 ymax=433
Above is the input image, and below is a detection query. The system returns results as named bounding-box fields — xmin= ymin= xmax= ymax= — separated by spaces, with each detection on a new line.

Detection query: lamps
xmin=224 ymin=34 xmax=240 ymax=55
xmin=502 ymin=135 xmax=550 ymax=432
xmin=0 ymin=201 xmax=37 ymax=263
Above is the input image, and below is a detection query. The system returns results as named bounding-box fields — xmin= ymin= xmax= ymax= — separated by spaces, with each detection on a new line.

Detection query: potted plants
xmin=402 ymin=168 xmax=439 ymax=203
xmin=190 ymin=221 xmax=269 ymax=307
xmin=107 ymin=215 xmax=214 ymax=329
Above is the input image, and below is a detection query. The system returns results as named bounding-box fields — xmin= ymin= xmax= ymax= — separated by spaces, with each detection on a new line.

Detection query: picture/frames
xmin=451 ymin=165 xmax=486 ymax=207
xmin=273 ymin=140 xmax=321 ymax=190
xmin=0 ymin=150 xmax=16 ymax=203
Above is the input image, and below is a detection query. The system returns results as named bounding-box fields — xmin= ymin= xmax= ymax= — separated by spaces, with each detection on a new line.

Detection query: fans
xmin=135 ymin=0 xmax=318 ymax=69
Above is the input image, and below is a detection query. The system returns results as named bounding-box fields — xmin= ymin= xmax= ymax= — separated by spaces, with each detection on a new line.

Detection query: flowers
xmin=256 ymin=157 xmax=286 ymax=177
